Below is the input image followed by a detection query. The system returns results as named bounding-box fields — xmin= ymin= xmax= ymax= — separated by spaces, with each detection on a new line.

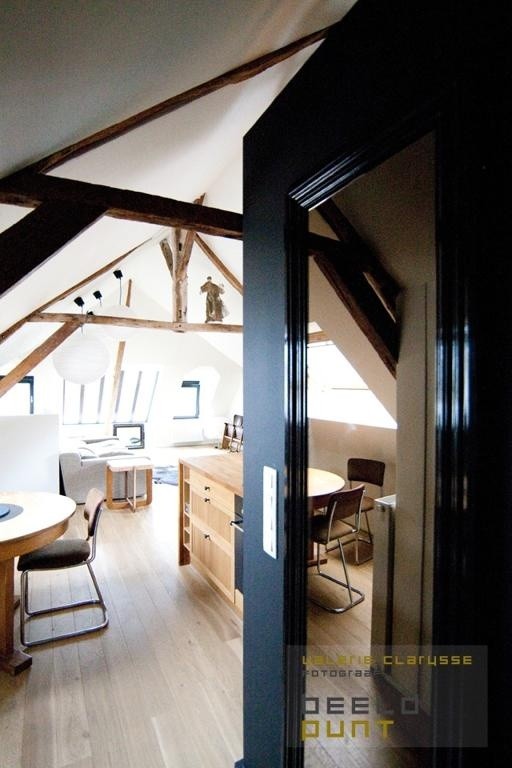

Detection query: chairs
xmin=325 ymin=458 xmax=386 ymax=565
xmin=306 ymin=484 xmax=365 ymax=614
xmin=17 ymin=488 xmax=109 ymax=648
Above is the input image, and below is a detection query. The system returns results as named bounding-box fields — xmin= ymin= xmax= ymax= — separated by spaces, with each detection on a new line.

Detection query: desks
xmin=105 ymin=458 xmax=153 ymax=512
xmin=178 ymin=452 xmax=346 ymax=622
xmin=0 ymin=489 xmax=76 ymax=677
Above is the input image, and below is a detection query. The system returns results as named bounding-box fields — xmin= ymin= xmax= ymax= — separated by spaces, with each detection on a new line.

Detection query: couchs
xmin=59 ymin=436 xmax=150 ymax=505
